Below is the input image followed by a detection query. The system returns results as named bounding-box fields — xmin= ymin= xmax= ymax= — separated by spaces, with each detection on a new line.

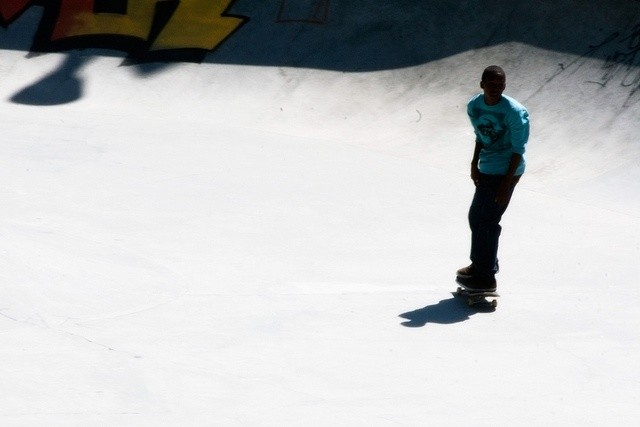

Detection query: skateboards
xmin=456 ymin=286 xmax=499 ymax=309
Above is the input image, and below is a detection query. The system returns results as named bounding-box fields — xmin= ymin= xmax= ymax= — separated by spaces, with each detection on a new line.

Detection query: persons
xmin=456 ymin=63 xmax=530 ymax=292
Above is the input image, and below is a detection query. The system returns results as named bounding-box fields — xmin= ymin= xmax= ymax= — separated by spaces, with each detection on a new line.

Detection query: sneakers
xmin=457 ymin=274 xmax=496 ymax=292
xmin=457 ymin=260 xmax=499 ymax=277
